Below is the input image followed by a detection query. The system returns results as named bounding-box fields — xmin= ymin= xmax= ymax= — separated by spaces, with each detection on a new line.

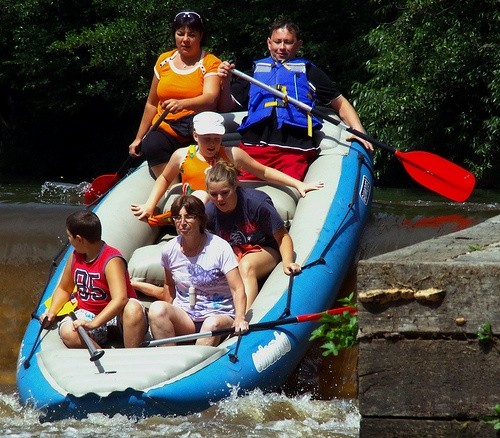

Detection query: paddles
xmin=139 ymin=305 xmax=357 ymax=348
xmin=225 ymin=59 xmax=476 ymax=203
xmin=84 ymin=109 xmax=169 ymax=206
xmin=44 ymin=288 xmax=105 ymax=361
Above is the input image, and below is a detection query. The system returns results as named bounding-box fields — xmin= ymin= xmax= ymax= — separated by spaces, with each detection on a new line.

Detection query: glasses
xmin=174 ymin=11 xmax=201 ymax=24
xmin=172 ymin=214 xmax=200 ymax=223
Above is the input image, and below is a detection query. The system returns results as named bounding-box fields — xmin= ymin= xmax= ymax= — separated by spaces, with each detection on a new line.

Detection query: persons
xmin=128 ymin=11 xmax=224 ymax=190
xmin=202 ymin=162 xmax=301 ymax=314
xmin=130 ymin=112 xmax=324 ymax=220
xmin=218 ymin=20 xmax=375 ymax=181
xmin=40 ymin=210 xmax=147 ymax=349
xmin=129 ymin=195 xmax=249 ymax=347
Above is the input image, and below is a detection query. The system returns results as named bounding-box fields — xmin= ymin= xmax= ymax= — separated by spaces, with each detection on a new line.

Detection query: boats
xmin=14 ymin=108 xmax=375 ymax=420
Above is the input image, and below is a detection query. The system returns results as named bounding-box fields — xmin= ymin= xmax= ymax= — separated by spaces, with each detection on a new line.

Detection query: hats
xmin=193 ymin=111 xmax=226 ymax=135
